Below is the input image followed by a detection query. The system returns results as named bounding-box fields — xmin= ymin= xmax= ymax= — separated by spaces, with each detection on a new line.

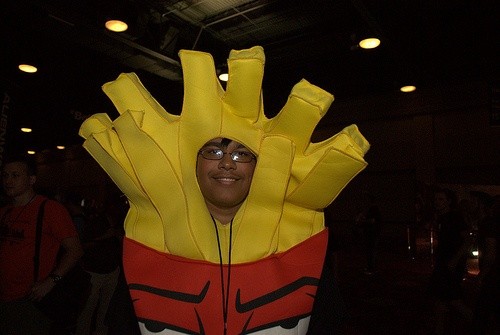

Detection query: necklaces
xmin=1 ymin=195 xmax=35 ymax=235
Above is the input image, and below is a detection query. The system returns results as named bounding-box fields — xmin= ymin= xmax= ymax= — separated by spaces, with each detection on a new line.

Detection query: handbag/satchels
xmin=30 ymin=260 xmax=95 ymax=328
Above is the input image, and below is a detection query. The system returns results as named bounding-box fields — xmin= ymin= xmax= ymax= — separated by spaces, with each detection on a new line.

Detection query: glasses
xmin=199 ymin=148 xmax=255 ymax=163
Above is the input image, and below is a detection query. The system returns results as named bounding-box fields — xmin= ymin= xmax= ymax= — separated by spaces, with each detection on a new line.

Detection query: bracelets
xmin=48 ymin=273 xmax=63 ymax=282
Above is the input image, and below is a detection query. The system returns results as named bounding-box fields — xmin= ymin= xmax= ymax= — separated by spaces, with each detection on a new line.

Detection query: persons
xmin=196 ymin=137 xmax=257 ymax=225
xmin=424 ymin=187 xmax=500 ymax=335
xmin=41 ymin=190 xmax=120 ymax=335
xmin=0 ymin=153 xmax=85 ymax=335
